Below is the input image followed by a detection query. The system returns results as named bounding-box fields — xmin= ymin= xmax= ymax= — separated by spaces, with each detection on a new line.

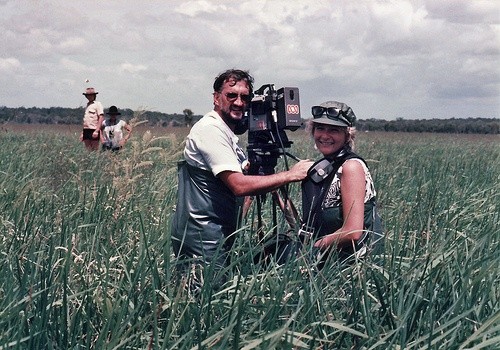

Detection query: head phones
xmin=307 ymin=144 xmax=352 ymax=184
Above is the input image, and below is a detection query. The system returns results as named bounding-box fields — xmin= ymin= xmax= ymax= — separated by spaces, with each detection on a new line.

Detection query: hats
xmin=311 ymin=100 xmax=356 ymax=127
xmin=83 ymin=88 xmax=99 ymax=95
xmin=105 ymin=106 xmax=121 ymax=115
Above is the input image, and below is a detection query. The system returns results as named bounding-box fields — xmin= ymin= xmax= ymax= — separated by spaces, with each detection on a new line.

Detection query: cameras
xmin=104 ymin=141 xmax=112 ymax=150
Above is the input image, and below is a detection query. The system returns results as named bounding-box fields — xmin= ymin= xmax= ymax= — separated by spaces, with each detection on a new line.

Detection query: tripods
xmin=237 ymin=144 xmax=304 ymax=236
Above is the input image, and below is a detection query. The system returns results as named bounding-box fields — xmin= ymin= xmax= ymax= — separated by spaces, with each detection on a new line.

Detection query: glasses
xmin=311 ymin=106 xmax=352 ymax=127
xmin=217 ymin=91 xmax=254 ymax=104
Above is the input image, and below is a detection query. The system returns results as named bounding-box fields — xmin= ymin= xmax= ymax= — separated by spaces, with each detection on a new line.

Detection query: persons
xmin=80 ymin=86 xmax=132 ymax=153
xmin=301 ymin=102 xmax=377 ymax=265
xmin=171 ymin=69 xmax=317 ymax=280
